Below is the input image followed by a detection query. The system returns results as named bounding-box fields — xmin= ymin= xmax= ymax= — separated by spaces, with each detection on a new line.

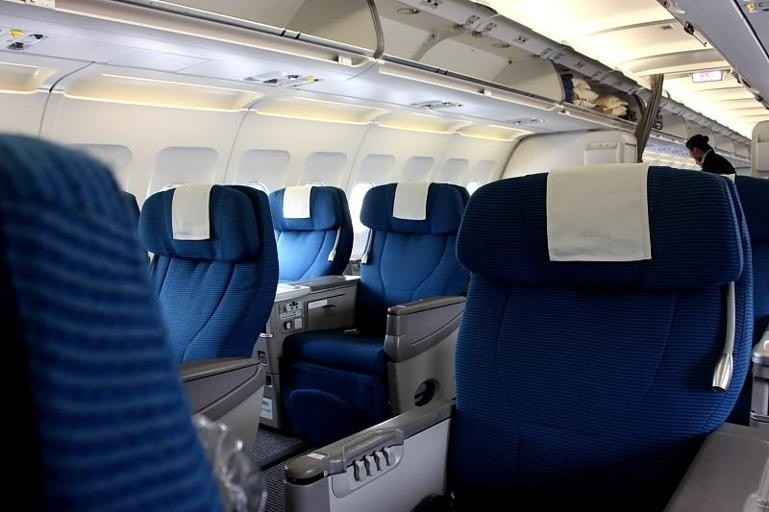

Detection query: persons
xmin=686 ymin=133 xmax=737 ymax=176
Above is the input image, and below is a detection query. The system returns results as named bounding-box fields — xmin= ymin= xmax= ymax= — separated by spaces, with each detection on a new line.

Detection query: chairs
xmin=0 ymin=131 xmax=768 ymax=512
